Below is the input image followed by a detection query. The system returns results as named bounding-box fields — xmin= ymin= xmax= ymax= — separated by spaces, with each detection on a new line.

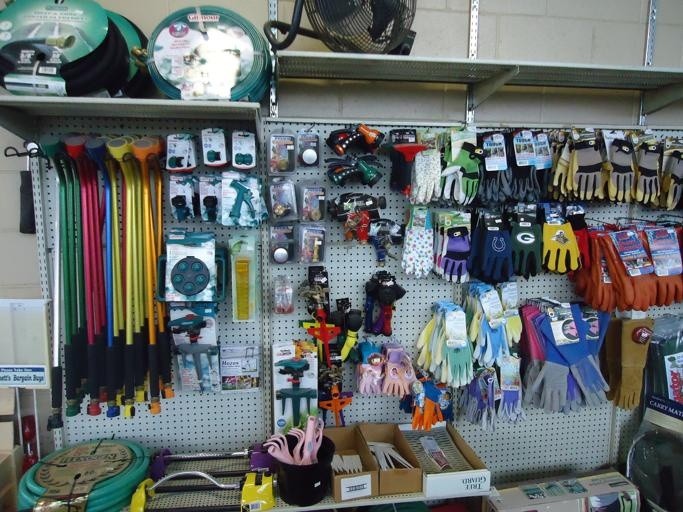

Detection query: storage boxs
xmin=399 ymin=424 xmax=491 ymax=496
xmin=321 ymin=423 xmax=377 ymax=503
xmin=358 ymin=423 xmax=420 ymax=495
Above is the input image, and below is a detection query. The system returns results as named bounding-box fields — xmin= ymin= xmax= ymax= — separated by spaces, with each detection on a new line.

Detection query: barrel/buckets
xmin=264 ymin=432 xmax=334 ymax=506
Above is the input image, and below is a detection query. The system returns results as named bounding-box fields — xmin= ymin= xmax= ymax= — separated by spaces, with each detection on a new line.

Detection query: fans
xmin=265 ymin=0 xmax=418 ymax=61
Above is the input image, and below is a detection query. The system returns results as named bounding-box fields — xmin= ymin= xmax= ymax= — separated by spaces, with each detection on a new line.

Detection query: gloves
xmin=568 ymin=219 xmax=683 ymax=315
xmin=399 ymin=282 xmax=525 ymax=432
xmin=353 ymin=343 xmax=414 ymax=397
xmin=518 ymin=304 xmax=609 ymax=414
xmin=605 ymin=315 xmax=656 ymax=414
xmin=408 ymin=129 xmax=683 ymax=208
xmin=398 ymin=204 xmax=589 ymax=285
